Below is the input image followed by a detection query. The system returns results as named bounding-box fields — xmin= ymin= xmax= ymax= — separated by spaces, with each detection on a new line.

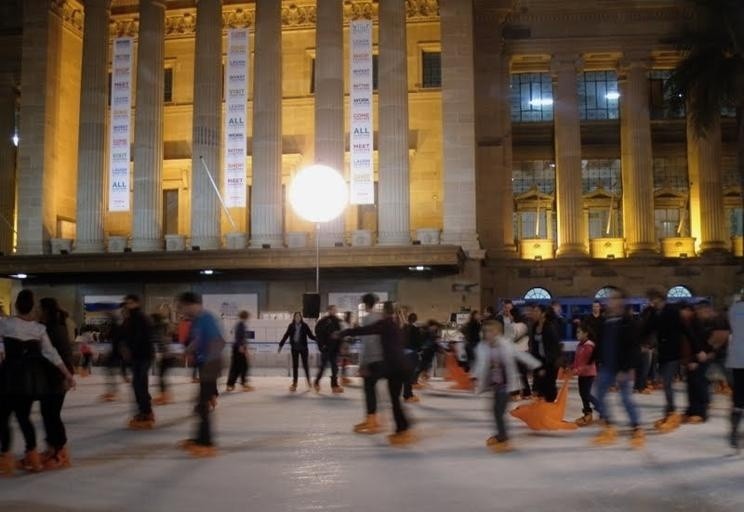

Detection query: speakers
xmin=303 ymin=292 xmax=320 ymax=318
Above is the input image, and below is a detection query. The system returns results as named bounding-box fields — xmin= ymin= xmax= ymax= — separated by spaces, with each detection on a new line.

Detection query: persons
xmin=1 ymin=291 xmax=256 ymax=476
xmin=280 ymin=290 xmax=744 ymax=445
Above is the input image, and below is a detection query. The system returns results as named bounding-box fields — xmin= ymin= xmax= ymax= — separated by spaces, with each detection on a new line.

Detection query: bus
xmin=494 ymin=298 xmax=715 ymax=366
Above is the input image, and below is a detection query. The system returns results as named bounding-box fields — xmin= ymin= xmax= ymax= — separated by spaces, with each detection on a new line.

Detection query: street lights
xmin=287 ymin=162 xmax=353 ymax=327
